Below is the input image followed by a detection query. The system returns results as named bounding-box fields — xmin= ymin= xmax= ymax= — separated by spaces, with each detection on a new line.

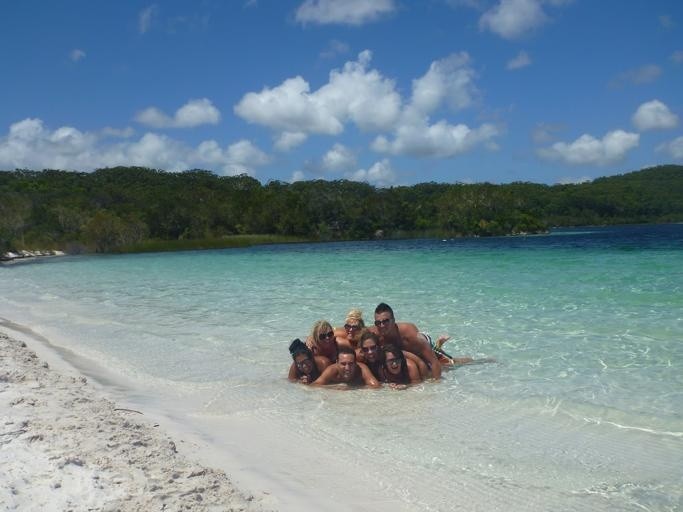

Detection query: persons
xmin=284 ymin=301 xmax=495 ymax=391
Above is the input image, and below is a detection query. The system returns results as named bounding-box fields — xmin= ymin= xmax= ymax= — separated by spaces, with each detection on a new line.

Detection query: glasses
xmin=362 ymin=346 xmax=376 ymax=352
xmin=386 ymin=358 xmax=402 ymax=365
xmin=344 ymin=324 xmax=359 ymax=331
xmin=319 ymin=331 xmax=334 ymax=340
xmin=296 ymin=359 xmax=312 ymax=368
xmin=375 ymin=319 xmax=389 ymax=326
